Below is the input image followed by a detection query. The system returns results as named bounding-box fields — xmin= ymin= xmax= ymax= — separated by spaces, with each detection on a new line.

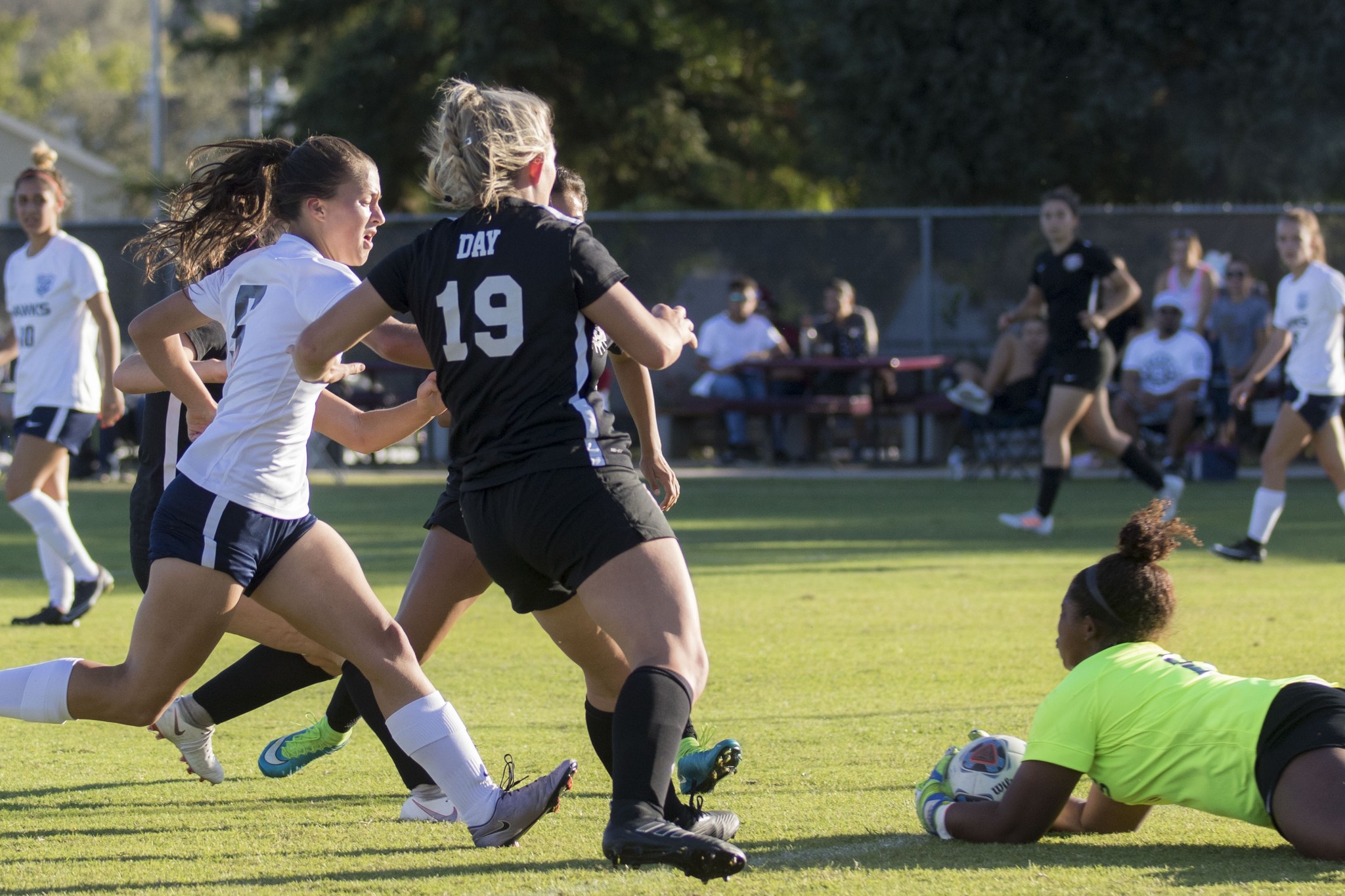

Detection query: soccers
xmin=949 ymin=733 xmax=1029 ymax=803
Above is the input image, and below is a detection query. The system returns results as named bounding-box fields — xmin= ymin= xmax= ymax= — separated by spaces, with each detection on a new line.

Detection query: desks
xmin=718 ymin=356 xmax=948 ymax=467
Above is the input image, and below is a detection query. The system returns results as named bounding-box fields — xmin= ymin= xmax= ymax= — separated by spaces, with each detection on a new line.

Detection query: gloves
xmin=941 ymin=728 xmax=991 ymax=801
xmin=914 ymin=753 xmax=956 ymax=841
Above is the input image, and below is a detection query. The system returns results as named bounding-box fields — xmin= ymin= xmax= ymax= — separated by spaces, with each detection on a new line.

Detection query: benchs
xmin=653 ymin=390 xmax=874 ymax=468
xmin=719 ymin=390 xmax=963 ymax=468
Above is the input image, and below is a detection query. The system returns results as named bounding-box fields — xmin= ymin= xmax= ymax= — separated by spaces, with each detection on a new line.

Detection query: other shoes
xmin=945 ymin=385 xmax=993 ymax=415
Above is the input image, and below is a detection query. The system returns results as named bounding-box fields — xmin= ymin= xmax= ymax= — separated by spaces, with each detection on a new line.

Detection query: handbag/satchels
xmin=1187 ymin=443 xmax=1240 ymax=481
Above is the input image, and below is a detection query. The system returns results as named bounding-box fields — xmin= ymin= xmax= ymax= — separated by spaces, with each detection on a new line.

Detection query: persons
xmin=288 ymin=77 xmax=747 ymax=885
xmin=262 ymin=164 xmax=744 ymax=796
xmin=112 ymin=241 xmax=464 ymax=826
xmin=689 ymin=190 xmax=1345 ymax=565
xmin=908 ymin=496 xmax=1344 ymax=858
xmin=1 ymin=141 xmax=128 ymax=626
xmin=0 ymin=133 xmax=579 ymax=848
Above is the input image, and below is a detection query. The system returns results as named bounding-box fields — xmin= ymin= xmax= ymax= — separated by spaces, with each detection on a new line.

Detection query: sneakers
xmin=467 ymin=759 xmax=579 ymax=848
xmin=676 ymin=738 xmax=742 ymax=795
xmin=12 ymin=602 xmax=74 ymax=624
xmin=258 ymin=715 xmax=353 ymax=779
xmin=671 ymin=803 xmax=740 ymax=843
xmin=1210 ymin=539 xmax=1263 ymax=562
xmin=66 ymin=563 xmax=116 ymax=624
xmin=146 ymin=696 xmax=225 ymax=786
xmin=602 ymin=814 xmax=748 ymax=884
xmin=999 ymin=507 xmax=1054 ymax=536
xmin=1156 ymin=475 xmax=1184 ymax=522
xmin=399 ymin=795 xmax=465 ymax=822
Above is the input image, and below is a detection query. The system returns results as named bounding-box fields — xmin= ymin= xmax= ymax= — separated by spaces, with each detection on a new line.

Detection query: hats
xmin=1153 ymin=290 xmax=1187 ymax=315
